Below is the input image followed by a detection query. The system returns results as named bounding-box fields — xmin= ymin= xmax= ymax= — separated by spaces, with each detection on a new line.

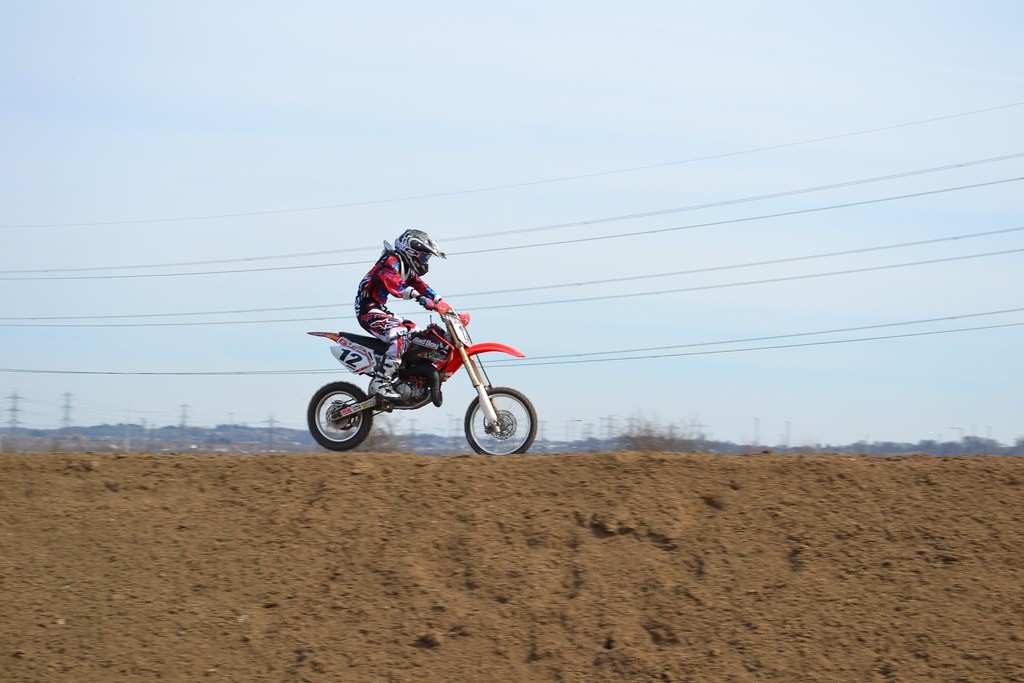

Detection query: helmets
xmin=395 ymin=229 xmax=446 ymax=276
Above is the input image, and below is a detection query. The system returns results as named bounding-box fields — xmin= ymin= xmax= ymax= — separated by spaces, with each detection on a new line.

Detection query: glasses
xmin=417 ymin=252 xmax=432 ymax=265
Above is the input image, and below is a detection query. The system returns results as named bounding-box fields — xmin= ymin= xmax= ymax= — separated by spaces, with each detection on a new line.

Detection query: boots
xmin=369 ymin=355 xmax=403 ymax=400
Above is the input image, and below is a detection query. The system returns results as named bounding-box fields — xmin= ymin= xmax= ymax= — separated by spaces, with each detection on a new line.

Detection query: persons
xmin=354 ymin=229 xmax=459 ymax=401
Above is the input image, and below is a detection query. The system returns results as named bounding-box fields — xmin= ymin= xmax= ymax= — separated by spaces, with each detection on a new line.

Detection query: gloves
xmin=420 ymin=296 xmax=435 ymax=311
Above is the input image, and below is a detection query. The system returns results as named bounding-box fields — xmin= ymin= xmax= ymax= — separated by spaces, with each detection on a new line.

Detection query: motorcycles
xmin=307 ymin=300 xmax=538 ymax=455
